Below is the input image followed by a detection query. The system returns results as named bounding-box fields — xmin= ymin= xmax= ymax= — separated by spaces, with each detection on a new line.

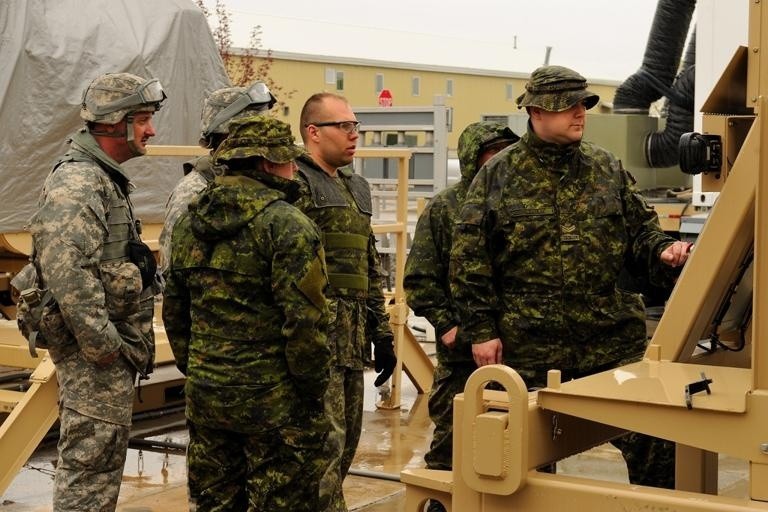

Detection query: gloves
xmin=374 ymin=339 xmax=398 ymax=387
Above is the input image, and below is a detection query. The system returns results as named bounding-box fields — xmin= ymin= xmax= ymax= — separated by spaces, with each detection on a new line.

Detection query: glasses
xmin=140 ymin=77 xmax=167 ymax=104
xmin=307 ymin=120 xmax=362 ymax=135
xmin=239 ymin=82 xmax=281 ymax=107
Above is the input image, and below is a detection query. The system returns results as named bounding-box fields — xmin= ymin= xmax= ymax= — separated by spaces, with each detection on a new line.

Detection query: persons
xmin=283 ymin=91 xmax=400 ymax=511
xmin=8 ymin=67 xmax=168 ymax=510
xmin=157 ymin=80 xmax=281 ymax=277
xmin=399 ymin=120 xmax=522 ymax=512
xmin=158 ymin=110 xmax=336 ymax=509
xmin=445 ymin=64 xmax=698 ymax=491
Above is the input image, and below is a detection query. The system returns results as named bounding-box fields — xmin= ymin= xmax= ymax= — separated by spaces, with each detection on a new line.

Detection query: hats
xmin=211 ymin=117 xmax=311 ymax=164
xmin=513 ymin=64 xmax=599 ymax=110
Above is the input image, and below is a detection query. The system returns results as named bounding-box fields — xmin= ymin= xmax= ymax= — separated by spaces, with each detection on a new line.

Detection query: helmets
xmin=79 ymin=73 xmax=155 ymax=122
xmin=200 ymin=85 xmax=278 ymax=143
